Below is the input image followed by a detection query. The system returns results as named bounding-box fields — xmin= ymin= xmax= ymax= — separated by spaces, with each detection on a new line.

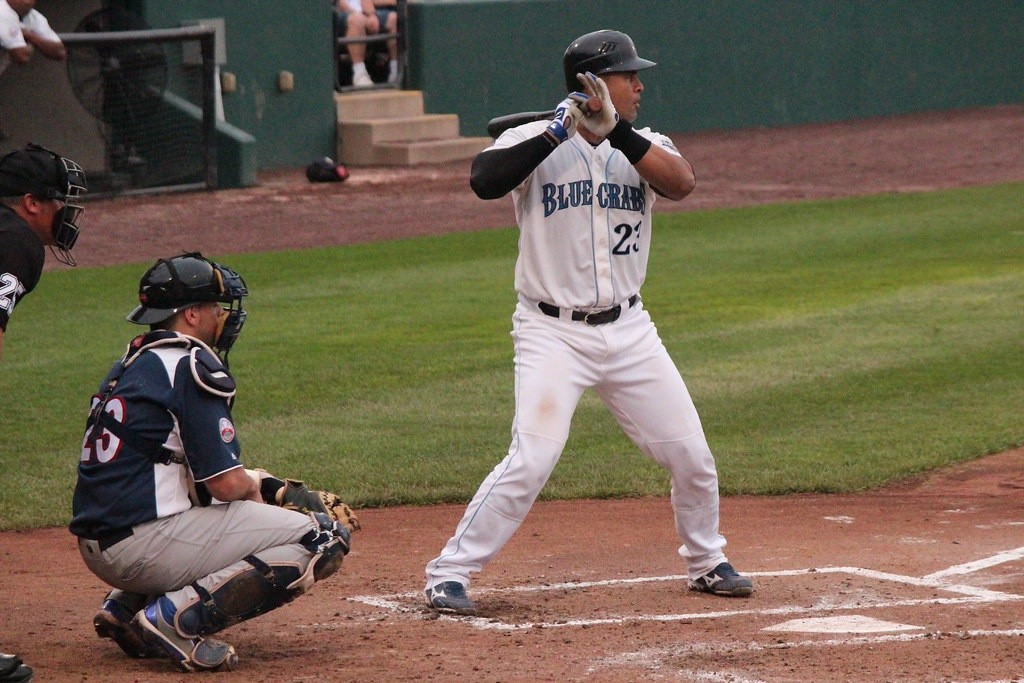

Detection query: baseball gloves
xmin=280 ymin=478 xmax=363 ymax=536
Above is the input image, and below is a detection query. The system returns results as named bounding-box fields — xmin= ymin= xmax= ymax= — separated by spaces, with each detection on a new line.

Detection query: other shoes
xmin=388 ymin=72 xmax=399 ymax=83
xmin=352 ymin=71 xmax=375 ymax=89
xmin=0 ymin=653 xmax=35 ymax=683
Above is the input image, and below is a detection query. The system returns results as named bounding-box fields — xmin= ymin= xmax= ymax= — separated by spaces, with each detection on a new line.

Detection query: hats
xmin=1 ymin=144 xmax=79 ymax=198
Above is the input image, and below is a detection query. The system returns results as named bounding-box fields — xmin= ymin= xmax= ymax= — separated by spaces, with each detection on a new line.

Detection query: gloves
xmin=547 ymin=71 xmax=619 ymax=145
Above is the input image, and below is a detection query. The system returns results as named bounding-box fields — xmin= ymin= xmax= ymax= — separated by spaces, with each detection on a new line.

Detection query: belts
xmin=538 ymin=295 xmax=639 ymax=326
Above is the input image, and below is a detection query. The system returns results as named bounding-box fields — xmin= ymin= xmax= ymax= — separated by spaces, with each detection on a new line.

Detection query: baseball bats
xmin=486 ymin=96 xmax=604 ymax=139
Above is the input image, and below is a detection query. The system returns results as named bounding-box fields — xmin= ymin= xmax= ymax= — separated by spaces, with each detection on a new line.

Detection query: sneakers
xmin=686 ymin=561 xmax=751 ymax=595
xmin=424 ymin=582 xmax=473 ymax=615
xmin=92 ymin=588 xmax=167 ymax=659
xmin=130 ymin=597 xmax=238 ymax=673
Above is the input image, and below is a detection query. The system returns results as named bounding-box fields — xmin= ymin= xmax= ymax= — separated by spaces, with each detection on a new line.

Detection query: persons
xmin=337 ymin=0 xmax=398 ymax=86
xmin=69 ymin=250 xmax=360 ymax=673
xmin=0 ymin=0 xmax=66 ymax=72
xmin=422 ymin=29 xmax=754 ymax=613
xmin=0 ymin=144 xmax=88 ymax=681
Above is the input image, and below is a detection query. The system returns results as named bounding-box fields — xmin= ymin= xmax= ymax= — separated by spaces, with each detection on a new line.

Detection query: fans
xmin=66 ymin=8 xmax=168 ymax=173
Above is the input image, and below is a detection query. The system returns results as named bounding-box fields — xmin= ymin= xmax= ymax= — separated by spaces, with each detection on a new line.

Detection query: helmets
xmin=124 ymin=253 xmax=226 ymax=325
xmin=563 ymin=28 xmax=657 ymax=94
